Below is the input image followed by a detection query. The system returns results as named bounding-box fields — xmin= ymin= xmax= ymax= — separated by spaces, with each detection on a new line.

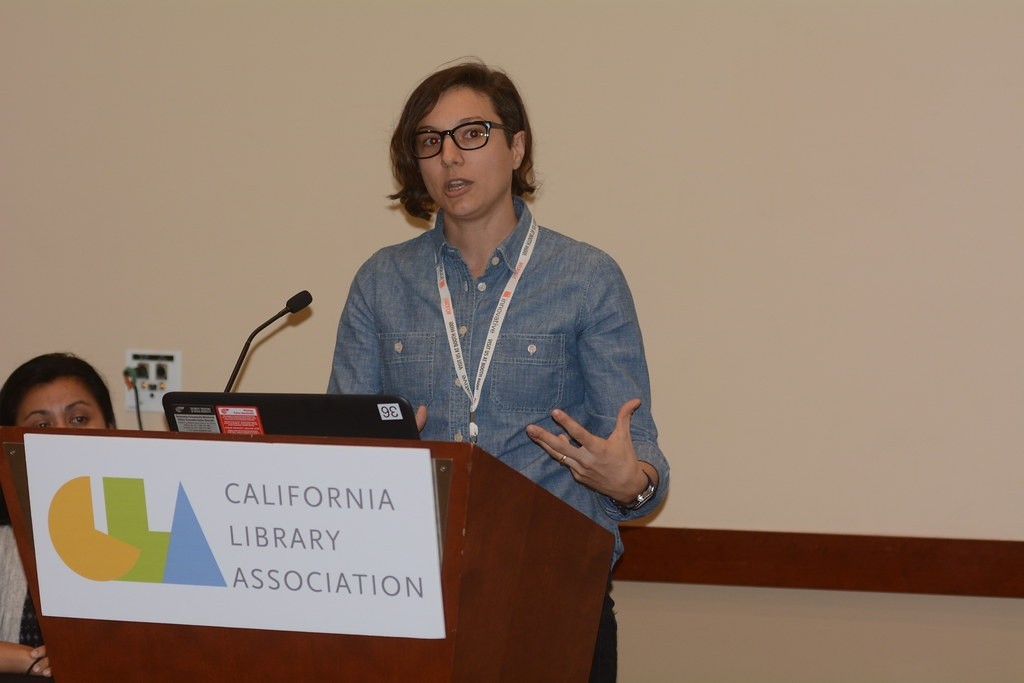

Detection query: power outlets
xmin=124 ymin=349 xmax=181 ymax=413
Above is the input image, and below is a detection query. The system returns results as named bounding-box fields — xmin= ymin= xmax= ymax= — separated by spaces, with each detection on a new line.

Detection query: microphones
xmin=224 ymin=290 xmax=313 ymax=393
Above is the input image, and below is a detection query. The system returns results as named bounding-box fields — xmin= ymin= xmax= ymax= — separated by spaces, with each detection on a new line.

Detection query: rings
xmin=560 ymin=455 xmax=566 ymax=464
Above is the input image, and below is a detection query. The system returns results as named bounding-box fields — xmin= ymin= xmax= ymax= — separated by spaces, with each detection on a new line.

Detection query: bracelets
xmin=27 ymin=657 xmax=43 ymax=673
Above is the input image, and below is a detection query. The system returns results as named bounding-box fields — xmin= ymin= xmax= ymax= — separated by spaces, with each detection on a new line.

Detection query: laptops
xmin=163 ymin=392 xmax=422 ymax=440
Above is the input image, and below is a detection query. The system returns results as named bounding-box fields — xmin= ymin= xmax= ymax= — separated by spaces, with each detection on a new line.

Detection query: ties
xmin=18 ymin=584 xmax=44 ymax=647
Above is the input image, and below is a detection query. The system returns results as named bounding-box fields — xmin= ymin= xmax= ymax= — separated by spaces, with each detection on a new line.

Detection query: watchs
xmin=611 ymin=469 xmax=655 ymax=513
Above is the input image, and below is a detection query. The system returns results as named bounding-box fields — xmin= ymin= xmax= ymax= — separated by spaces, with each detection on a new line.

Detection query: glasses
xmin=410 ymin=121 xmax=517 ymax=159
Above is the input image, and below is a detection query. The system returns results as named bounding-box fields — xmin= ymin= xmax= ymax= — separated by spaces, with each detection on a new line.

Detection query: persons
xmin=0 ymin=351 xmax=117 ymax=683
xmin=327 ymin=56 xmax=671 ymax=683
